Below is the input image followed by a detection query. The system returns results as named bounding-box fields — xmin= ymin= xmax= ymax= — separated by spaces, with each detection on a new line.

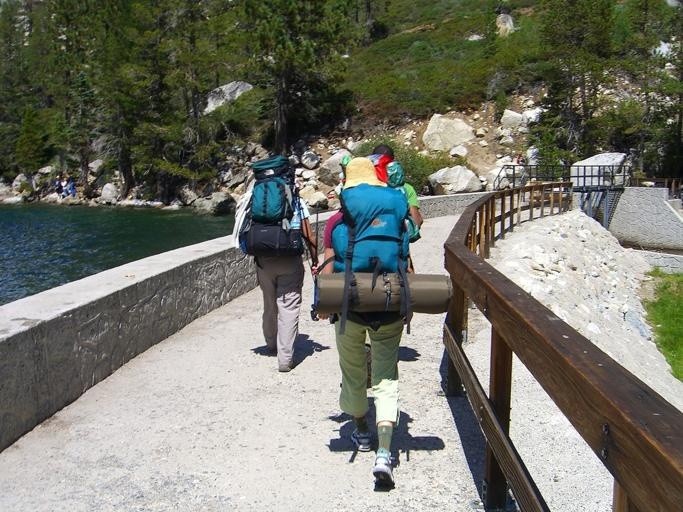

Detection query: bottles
xmin=289 ymin=212 xmax=303 ymax=249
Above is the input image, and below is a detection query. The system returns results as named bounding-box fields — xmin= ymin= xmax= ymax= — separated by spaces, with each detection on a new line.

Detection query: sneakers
xmin=373 ymin=455 xmax=396 ymax=487
xmin=352 ymin=430 xmax=372 ymax=452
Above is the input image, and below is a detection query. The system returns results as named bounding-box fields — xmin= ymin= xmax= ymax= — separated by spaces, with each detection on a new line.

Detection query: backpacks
xmin=331 ymin=187 xmax=406 ymax=328
xmin=363 ymin=153 xmax=419 ymax=241
xmin=250 ymin=157 xmax=293 ymax=222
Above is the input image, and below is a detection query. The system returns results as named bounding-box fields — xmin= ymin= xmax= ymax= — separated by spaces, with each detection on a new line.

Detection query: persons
xmin=372 ymin=144 xmax=423 ymax=228
xmin=320 ymin=158 xmax=414 ymax=487
xmin=55 ymin=175 xmax=75 ymax=198
xmin=254 ymin=188 xmax=320 ymax=372
